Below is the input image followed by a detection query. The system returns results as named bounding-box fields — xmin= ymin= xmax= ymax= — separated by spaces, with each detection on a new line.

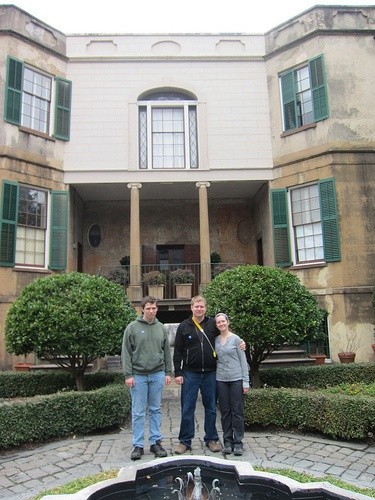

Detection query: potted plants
xmin=143 ymin=270 xmax=167 ymax=299
xmin=169 ymin=268 xmax=194 ymax=298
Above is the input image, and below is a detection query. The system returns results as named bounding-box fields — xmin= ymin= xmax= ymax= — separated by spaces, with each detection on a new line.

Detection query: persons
xmin=173 ymin=295 xmax=246 ymax=454
xmin=215 ymin=313 xmax=249 ymax=456
xmin=121 ymin=295 xmax=172 ymax=460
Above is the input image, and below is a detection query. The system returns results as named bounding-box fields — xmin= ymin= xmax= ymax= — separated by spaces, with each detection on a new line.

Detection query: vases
xmin=14 ymin=363 xmax=33 ymax=372
xmin=338 ymin=352 xmax=356 ymax=364
xmin=310 ymin=354 xmax=327 ymax=364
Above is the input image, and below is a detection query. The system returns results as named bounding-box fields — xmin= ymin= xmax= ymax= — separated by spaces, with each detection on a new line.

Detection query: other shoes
xmin=175 ymin=442 xmax=189 ymax=454
xmin=233 ymin=447 xmax=243 ymax=455
xmin=131 ymin=447 xmax=144 ymax=460
xmin=224 ymin=447 xmax=232 ymax=454
xmin=150 ymin=444 xmax=167 ymax=457
xmin=207 ymin=439 xmax=222 ymax=452
